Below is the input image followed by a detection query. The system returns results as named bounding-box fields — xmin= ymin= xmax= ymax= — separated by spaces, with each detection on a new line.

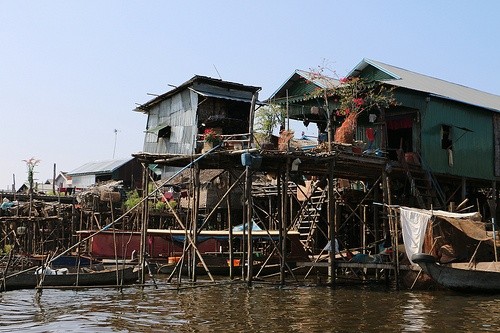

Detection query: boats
xmin=157 ymin=259 xmax=297 ymax=276
xmin=411 ymin=253 xmax=500 ymax=292
xmin=0 ymin=264 xmax=131 ymax=288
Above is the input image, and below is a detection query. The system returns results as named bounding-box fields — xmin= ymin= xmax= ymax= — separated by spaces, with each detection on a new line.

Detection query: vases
xmin=204 ymin=141 xmax=219 ymax=152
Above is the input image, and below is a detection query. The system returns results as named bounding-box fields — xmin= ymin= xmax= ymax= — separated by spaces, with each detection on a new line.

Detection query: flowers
xmin=202 ymin=128 xmax=221 ymax=142
xmin=299 ymin=56 xmax=403 ymax=119
